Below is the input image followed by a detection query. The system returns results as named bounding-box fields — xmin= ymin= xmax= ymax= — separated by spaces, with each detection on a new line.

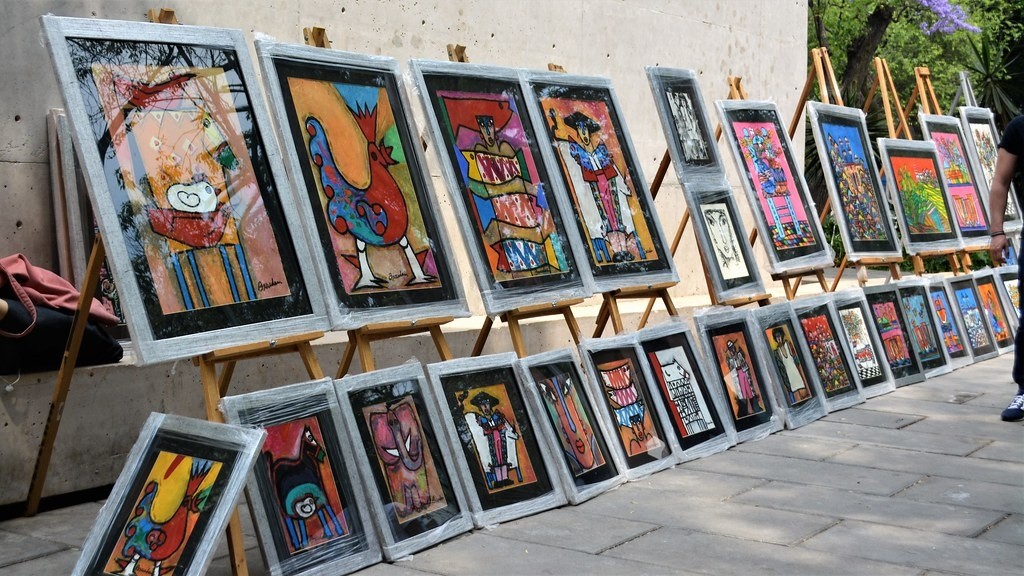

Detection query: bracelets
xmin=990 ymin=231 xmax=1004 ymax=237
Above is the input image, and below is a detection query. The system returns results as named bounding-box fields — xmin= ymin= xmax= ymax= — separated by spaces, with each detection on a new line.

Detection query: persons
xmin=990 ymin=115 xmax=1024 ymax=423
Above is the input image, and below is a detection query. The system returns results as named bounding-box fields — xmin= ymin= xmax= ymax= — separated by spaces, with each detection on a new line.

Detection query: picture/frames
xmin=41 ymin=14 xmax=1023 ymax=576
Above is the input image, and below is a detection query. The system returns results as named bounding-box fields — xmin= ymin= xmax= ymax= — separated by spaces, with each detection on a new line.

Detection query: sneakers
xmin=1001 ymin=390 xmax=1024 ymax=421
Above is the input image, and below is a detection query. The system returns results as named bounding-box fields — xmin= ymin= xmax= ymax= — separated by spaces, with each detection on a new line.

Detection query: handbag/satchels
xmin=0 ymin=253 xmax=121 ymax=339
